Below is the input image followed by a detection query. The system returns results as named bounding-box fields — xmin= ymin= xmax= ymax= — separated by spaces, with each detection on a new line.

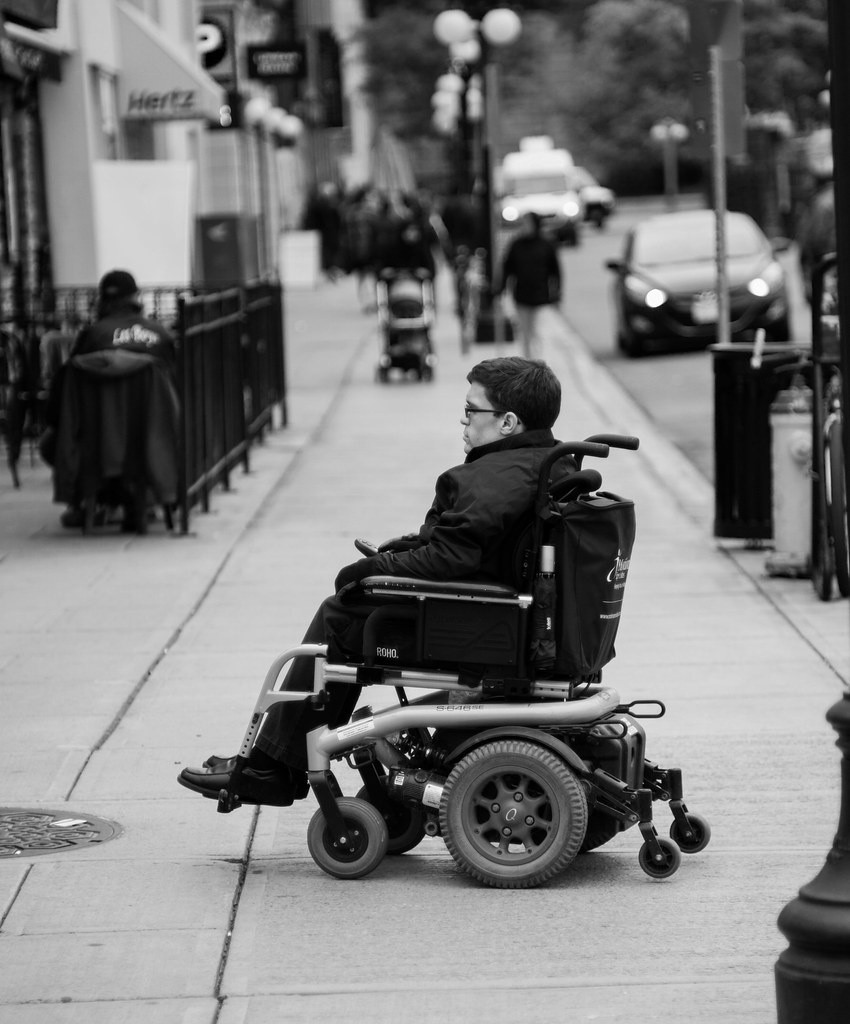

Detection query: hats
xmin=98 ymin=268 xmax=137 ymax=307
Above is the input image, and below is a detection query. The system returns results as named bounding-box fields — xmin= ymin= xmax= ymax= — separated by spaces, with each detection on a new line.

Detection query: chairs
xmin=1 ymin=328 xmax=73 ymax=488
xmin=66 ymin=367 xmax=178 ymax=535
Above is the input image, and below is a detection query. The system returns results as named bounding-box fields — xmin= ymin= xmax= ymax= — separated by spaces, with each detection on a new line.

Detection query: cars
xmin=602 ymin=209 xmax=795 ymax=359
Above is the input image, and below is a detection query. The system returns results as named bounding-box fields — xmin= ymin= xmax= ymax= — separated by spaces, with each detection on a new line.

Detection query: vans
xmin=572 ymin=165 xmax=615 ymax=225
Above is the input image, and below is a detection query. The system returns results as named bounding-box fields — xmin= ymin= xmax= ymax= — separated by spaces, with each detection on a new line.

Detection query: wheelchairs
xmin=207 ymin=435 xmax=712 ymax=888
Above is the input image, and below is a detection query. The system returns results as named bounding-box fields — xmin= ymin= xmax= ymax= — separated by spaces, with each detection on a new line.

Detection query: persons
xmin=39 ymin=271 xmax=176 ymax=534
xmin=178 ymin=356 xmax=579 ymax=807
xmin=314 ymin=184 xmax=489 ymax=379
xmin=494 ymin=212 xmax=562 ymax=354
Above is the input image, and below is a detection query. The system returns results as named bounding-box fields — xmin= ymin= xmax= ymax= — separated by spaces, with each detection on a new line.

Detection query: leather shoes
xmin=176 ymin=756 xmax=294 ymax=809
xmin=202 ymin=754 xmax=310 ymax=801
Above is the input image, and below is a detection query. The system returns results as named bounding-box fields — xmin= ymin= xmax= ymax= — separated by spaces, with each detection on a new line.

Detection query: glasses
xmin=462 ymin=406 xmax=521 ymax=426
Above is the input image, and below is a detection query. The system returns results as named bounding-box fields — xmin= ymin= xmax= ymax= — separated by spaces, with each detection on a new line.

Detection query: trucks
xmin=499 ymin=135 xmax=582 ymax=247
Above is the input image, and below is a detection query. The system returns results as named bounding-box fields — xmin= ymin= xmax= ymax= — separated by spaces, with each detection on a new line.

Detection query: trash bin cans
xmin=707 ymin=340 xmax=820 ymax=541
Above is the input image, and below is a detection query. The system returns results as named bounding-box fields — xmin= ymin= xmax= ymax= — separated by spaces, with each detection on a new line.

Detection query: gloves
xmin=334 ymin=560 xmax=367 ymax=608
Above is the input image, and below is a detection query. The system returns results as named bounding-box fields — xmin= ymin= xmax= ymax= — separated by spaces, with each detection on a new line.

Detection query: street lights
xmin=429 ymin=6 xmax=523 ymax=338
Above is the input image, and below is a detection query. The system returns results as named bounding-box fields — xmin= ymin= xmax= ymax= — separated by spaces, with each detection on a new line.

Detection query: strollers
xmin=376 ymin=265 xmax=437 ymax=384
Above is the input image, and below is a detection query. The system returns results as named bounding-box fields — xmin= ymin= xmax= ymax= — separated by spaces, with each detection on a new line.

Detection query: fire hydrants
xmin=761 ymin=374 xmax=816 ymax=579
